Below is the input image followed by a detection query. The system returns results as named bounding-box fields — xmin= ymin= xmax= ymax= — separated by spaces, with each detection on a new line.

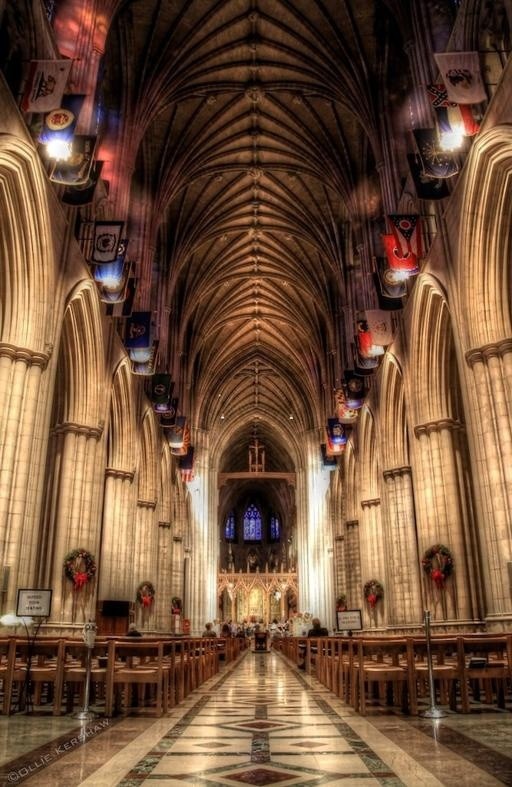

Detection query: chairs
xmin=277 ymin=634 xmax=512 ymax=716
xmin=0 ymin=636 xmax=245 ymax=717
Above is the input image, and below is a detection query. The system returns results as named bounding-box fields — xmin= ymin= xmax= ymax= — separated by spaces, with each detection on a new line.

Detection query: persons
xmin=203 ymin=617 xmax=289 ymax=638
xmin=297 ymin=618 xmax=329 ymax=669
xmin=126 ymin=622 xmax=142 ymax=637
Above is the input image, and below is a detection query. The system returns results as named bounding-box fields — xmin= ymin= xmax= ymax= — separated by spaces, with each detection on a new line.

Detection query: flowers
xmin=171 ymin=596 xmax=183 ymax=614
xmin=64 ymin=547 xmax=97 ymax=588
xmin=421 ymin=545 xmax=455 ymax=590
xmin=363 ymin=579 xmax=383 ymax=611
xmin=134 ymin=581 xmax=155 ymax=610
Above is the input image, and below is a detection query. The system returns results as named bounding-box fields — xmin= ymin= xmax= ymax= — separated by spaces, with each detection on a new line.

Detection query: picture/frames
xmin=16 ymin=588 xmax=52 ymax=617
xmin=336 ymin=610 xmax=363 ymax=631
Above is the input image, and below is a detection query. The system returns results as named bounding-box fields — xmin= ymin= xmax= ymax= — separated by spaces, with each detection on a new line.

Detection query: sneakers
xmin=297 ymin=662 xmax=305 ymax=669
